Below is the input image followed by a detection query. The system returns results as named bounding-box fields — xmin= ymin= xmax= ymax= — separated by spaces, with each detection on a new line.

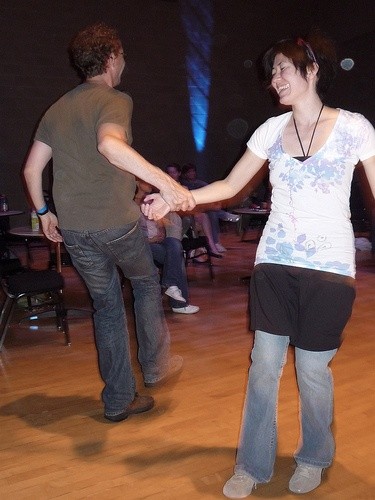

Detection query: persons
xmin=132 ymin=162 xmax=272 ymax=315
xmin=23 ymin=21 xmax=196 ymax=422
xmin=140 ymin=36 xmax=375 ymax=499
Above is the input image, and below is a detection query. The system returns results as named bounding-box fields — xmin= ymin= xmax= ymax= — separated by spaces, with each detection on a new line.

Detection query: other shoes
xmin=145 ymin=356 xmax=184 ymax=387
xmin=222 ymin=211 xmax=240 ymax=222
xmin=103 ymin=394 xmax=154 ymax=421
xmin=215 ymin=244 xmax=227 ymax=252
xmin=211 ymin=252 xmax=224 ymax=259
xmin=193 ymin=253 xmax=208 ymax=262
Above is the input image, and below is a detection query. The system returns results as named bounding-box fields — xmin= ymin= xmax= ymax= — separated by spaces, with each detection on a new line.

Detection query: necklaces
xmin=293 ymin=104 xmax=325 ymax=158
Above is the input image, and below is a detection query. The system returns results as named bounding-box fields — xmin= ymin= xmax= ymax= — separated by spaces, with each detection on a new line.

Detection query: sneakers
xmin=172 ymin=305 xmax=200 ymax=313
xmin=165 ymin=288 xmax=186 ymax=303
xmin=223 ymin=474 xmax=257 ymax=498
xmin=288 ymin=466 xmax=324 ymax=493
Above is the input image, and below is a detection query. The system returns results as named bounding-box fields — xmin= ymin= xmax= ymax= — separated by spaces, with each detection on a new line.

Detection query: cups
xmin=31 ymin=212 xmax=40 ymax=232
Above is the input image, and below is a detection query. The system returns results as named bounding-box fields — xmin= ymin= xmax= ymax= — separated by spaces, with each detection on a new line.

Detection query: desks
xmin=233 ymin=209 xmax=271 ymax=243
xmin=0 ymin=210 xmax=95 ymax=320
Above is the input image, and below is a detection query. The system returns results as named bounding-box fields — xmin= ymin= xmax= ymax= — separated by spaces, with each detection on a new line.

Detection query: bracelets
xmin=37 ymin=206 xmax=49 ymax=215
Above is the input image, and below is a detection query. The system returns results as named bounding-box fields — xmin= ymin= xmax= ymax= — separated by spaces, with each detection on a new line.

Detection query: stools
xmin=181 ymin=227 xmax=215 ymax=283
xmin=0 ymin=258 xmax=71 ymax=351
